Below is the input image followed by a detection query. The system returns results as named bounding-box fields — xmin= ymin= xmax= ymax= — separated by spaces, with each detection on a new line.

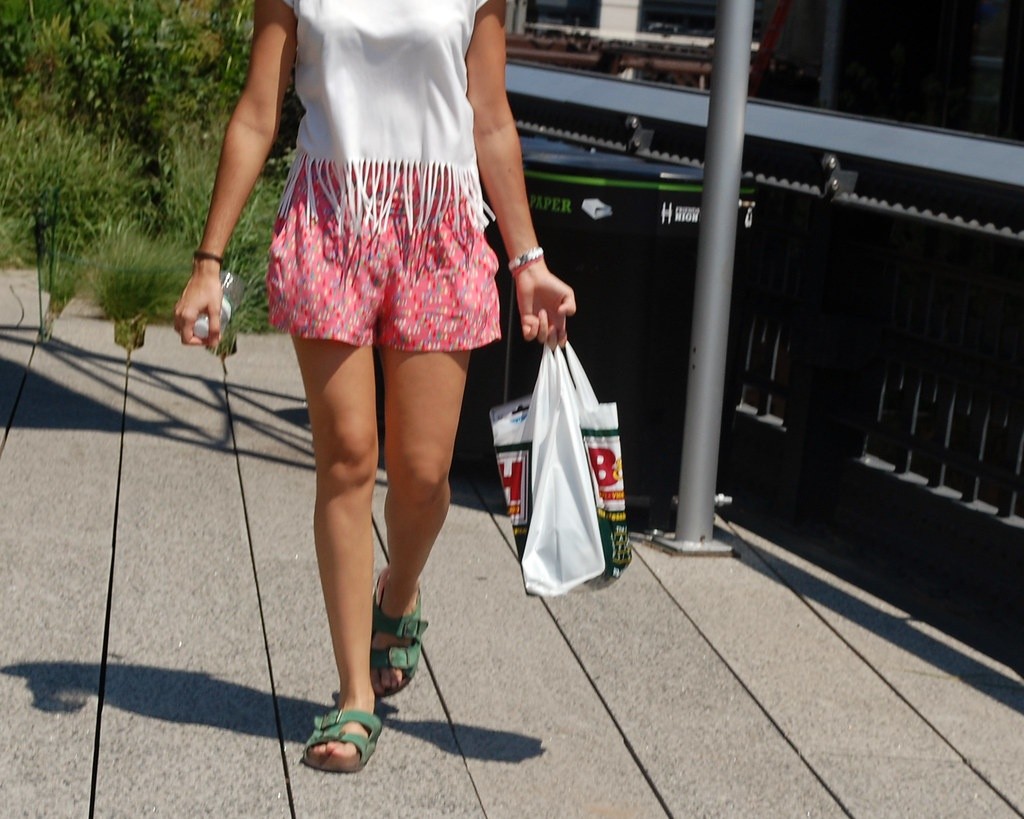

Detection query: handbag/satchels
xmin=489 ymin=338 xmax=632 ymax=598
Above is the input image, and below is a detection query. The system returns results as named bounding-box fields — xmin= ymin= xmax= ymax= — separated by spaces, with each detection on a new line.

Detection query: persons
xmin=175 ymin=0 xmax=576 ymax=774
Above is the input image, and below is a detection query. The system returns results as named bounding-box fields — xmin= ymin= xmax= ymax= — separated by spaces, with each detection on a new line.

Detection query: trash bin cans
xmin=497 ymin=132 xmax=759 ymax=521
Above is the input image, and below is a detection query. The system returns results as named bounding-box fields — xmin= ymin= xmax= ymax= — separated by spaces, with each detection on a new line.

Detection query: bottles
xmin=194 ymin=271 xmax=246 ymax=339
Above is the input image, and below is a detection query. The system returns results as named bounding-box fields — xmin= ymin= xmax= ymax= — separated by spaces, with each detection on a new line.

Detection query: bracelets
xmin=193 ymin=250 xmax=223 ymax=265
xmin=508 ymin=247 xmax=544 ymax=278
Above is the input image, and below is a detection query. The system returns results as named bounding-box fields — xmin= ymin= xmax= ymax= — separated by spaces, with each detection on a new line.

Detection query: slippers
xmin=368 ymin=567 xmax=429 ymax=697
xmin=301 ymin=707 xmax=383 ymax=773
